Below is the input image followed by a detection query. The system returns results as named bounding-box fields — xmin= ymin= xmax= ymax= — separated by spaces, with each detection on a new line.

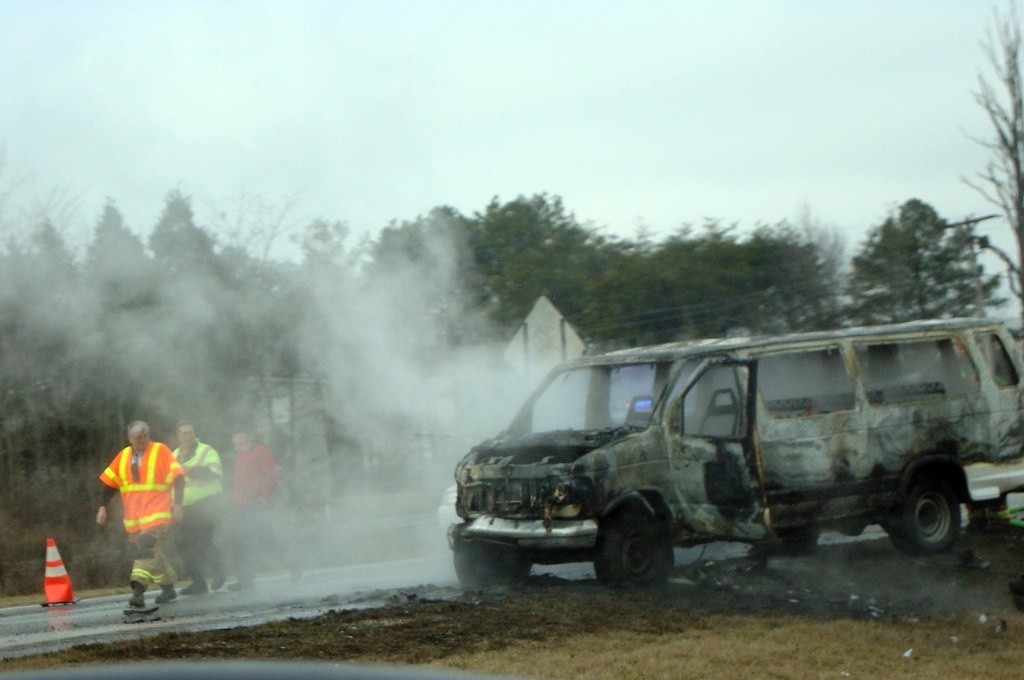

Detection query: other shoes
xmin=211 ymin=568 xmax=225 ymax=590
xmin=130 ymin=596 xmax=145 ymax=607
xmin=156 ymin=589 xmax=176 ymax=603
xmin=180 ymin=579 xmax=208 ymax=594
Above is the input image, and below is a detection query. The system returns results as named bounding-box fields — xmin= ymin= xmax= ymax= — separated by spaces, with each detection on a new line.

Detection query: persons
xmin=167 ymin=418 xmax=304 ymax=595
xmin=97 ymin=417 xmax=185 ymax=609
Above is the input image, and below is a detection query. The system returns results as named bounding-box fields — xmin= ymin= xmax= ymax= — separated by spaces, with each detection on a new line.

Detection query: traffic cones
xmin=40 ymin=538 xmax=81 ymax=607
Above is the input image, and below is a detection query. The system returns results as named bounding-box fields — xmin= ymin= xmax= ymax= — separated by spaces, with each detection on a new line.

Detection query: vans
xmin=445 ymin=319 xmax=1024 ymax=597
